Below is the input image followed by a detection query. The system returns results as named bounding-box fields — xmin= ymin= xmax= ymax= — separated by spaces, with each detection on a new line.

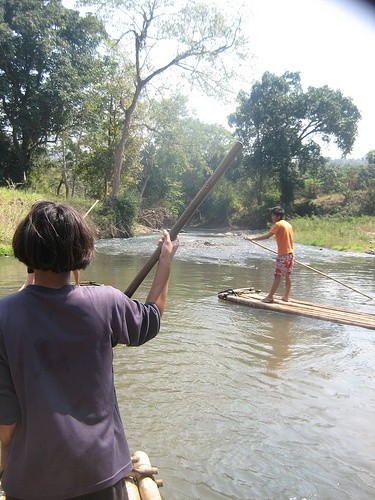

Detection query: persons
xmin=20 ymin=265 xmax=80 ymax=287
xmin=0 ymin=201 xmax=180 ymax=500
xmin=244 ymin=206 xmax=293 ymax=302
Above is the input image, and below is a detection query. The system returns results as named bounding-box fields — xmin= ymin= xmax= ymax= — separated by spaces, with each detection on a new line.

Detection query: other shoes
xmin=261 ymin=297 xmax=274 ymax=303
xmin=281 ymin=296 xmax=288 ymax=302
